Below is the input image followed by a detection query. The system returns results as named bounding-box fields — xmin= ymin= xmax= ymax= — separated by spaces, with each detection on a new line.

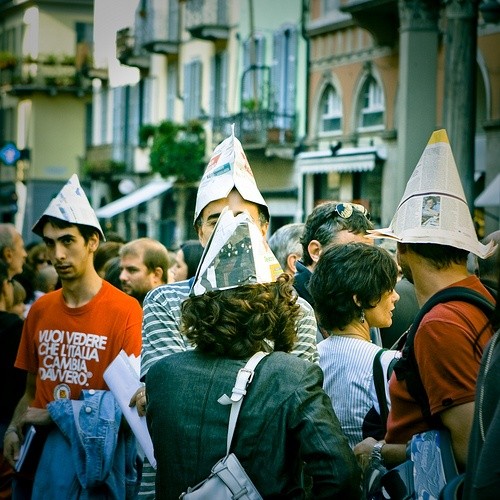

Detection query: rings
xmin=140 ymin=392 xmax=144 ymax=398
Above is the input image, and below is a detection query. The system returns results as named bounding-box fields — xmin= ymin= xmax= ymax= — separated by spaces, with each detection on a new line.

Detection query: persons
xmin=137 ymin=127 xmax=322 ymax=500
xmin=464 ymin=308 xmax=500 ymax=499
xmin=144 ymin=208 xmax=366 ymax=500
xmin=308 ymin=242 xmax=408 ymax=459
xmin=5 ymin=175 xmax=146 ymax=500
xmin=0 ymin=257 xmax=33 ymax=500
xmin=0 ymin=201 xmax=418 ymax=348
xmin=477 ymin=229 xmax=500 ymax=300
xmin=351 ymin=130 xmax=498 ymax=500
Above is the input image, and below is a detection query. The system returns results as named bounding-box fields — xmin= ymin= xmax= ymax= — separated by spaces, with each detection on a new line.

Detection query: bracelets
xmin=4 ymin=427 xmax=19 ymax=435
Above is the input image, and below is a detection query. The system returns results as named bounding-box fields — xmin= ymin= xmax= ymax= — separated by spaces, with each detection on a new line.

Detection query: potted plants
xmin=241 ymin=98 xmax=263 ymax=144
xmin=0 ymin=51 xmax=19 ymax=71
xmin=264 ymin=100 xmax=281 ymax=143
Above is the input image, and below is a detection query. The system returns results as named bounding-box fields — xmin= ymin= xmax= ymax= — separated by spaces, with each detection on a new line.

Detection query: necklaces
xmin=332 ymin=330 xmax=374 ymax=343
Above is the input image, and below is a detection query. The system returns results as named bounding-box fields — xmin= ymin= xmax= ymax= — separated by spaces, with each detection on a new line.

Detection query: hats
xmin=359 ymin=129 xmax=499 ymax=259
xmin=189 ymin=207 xmax=289 ymax=298
xmin=192 ymin=121 xmax=271 ymax=226
xmin=31 ymin=174 xmax=108 ymax=243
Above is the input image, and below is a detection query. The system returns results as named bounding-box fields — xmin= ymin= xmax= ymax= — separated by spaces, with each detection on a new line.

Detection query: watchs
xmin=370 ymin=440 xmax=387 ymax=466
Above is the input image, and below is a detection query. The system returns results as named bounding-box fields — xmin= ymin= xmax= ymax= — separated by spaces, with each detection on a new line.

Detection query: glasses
xmin=318 ymin=202 xmax=367 ymax=227
xmin=7 ymin=278 xmax=15 ymax=286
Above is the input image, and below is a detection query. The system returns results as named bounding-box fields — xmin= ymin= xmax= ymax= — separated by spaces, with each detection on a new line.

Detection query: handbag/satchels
xmin=180 ymin=454 xmax=264 ymax=500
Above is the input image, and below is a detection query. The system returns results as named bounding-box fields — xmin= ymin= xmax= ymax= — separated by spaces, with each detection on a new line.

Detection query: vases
xmin=285 ymin=128 xmax=295 ymax=143
